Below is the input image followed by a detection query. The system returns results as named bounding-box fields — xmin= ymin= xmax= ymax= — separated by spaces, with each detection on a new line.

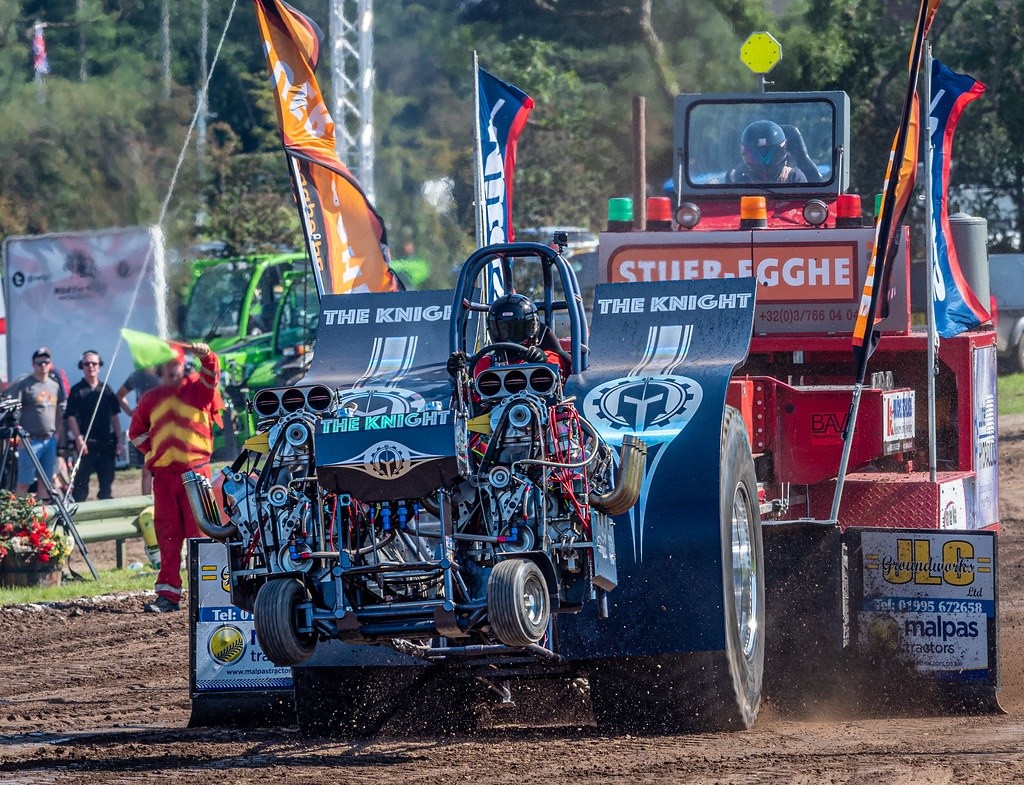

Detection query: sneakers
xmin=145 ymin=597 xmax=180 ymax=613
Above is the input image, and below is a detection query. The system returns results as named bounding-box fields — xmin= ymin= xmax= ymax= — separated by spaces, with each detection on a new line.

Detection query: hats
xmin=166 ymin=344 xmax=185 ymax=363
xmin=33 ymin=347 xmax=50 ymax=361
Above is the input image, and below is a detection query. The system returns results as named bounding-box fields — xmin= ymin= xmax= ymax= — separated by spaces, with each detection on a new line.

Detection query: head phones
xmin=78 ymin=351 xmax=103 ymax=370
xmin=154 ymin=362 xmax=192 ymax=378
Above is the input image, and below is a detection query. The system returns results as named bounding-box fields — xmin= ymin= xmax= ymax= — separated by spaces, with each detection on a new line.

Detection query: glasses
xmin=83 ymin=362 xmax=98 ymax=366
xmin=34 ymin=361 xmax=50 ymax=367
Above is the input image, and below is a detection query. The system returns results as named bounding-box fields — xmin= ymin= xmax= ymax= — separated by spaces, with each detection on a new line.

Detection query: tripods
xmin=0 ymin=413 xmax=101 ymax=581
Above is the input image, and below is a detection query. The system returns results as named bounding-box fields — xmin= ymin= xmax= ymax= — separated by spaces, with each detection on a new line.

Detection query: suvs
xmin=515 ymin=226 xmax=602 ymax=283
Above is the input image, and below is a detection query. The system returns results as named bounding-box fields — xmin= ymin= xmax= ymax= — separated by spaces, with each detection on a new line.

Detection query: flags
xmin=254 ymin=0 xmax=407 ymax=294
xmin=929 ymin=57 xmax=992 ymax=341
xmin=852 ymin=0 xmax=941 ymax=347
xmin=121 ymin=328 xmax=179 ymax=371
xmin=476 ymin=67 xmax=535 ymax=301
xmin=33 ymin=21 xmax=50 ymax=82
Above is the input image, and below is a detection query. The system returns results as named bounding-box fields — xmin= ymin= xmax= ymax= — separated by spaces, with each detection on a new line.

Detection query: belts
xmin=29 ymin=435 xmax=49 ymax=439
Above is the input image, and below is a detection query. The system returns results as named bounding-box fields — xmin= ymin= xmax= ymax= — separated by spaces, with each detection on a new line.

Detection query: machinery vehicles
xmin=180 ymin=31 xmax=1001 ymax=735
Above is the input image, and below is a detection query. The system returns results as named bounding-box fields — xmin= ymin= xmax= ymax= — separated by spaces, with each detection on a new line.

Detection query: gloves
xmin=525 ymin=346 xmax=547 ymax=363
xmin=447 ymin=352 xmax=466 ymax=377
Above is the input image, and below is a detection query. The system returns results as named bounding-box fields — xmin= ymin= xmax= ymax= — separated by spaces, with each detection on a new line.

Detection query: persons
xmin=129 ymin=340 xmax=226 ymax=614
xmin=10 ymin=348 xmax=162 ymax=503
xmin=446 ymin=295 xmax=564 ymax=453
xmin=709 ymin=120 xmax=823 ymax=184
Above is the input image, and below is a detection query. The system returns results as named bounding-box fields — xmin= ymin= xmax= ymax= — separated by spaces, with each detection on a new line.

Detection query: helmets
xmin=740 ymin=121 xmax=788 ymax=184
xmin=488 ymin=294 xmax=541 ymax=356
